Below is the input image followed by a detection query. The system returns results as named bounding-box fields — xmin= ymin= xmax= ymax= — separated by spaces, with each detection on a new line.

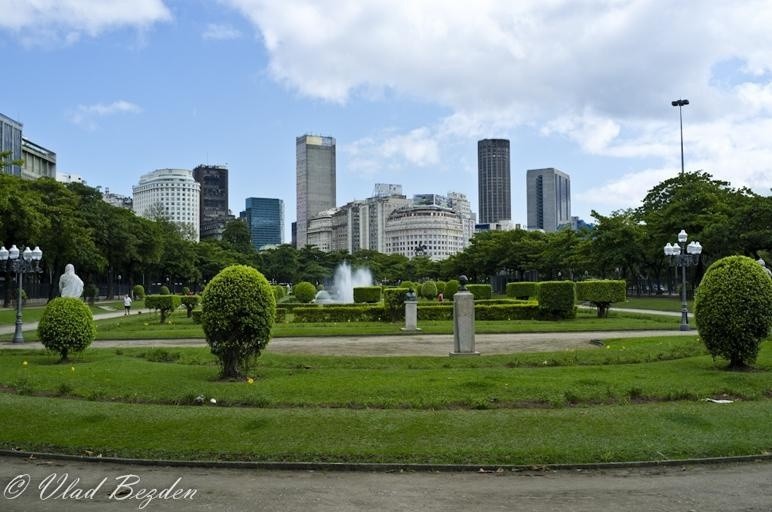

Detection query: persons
xmin=404 ymin=287 xmax=417 ymax=301
xmin=439 ymin=292 xmax=444 ymax=301
xmin=124 ymin=294 xmax=132 ymax=315
xmin=458 ymin=275 xmax=468 ymax=291
xmin=188 ymin=289 xmax=194 ymax=295
xmin=59 ymin=264 xmax=84 ymax=301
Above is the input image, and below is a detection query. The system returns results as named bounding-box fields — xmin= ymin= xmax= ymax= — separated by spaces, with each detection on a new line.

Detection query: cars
xmin=653 ymin=286 xmax=664 ymax=293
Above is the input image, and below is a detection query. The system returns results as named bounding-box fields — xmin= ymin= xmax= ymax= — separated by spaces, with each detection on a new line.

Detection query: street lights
xmin=118 ymin=272 xmax=122 ymax=301
xmin=670 ymin=99 xmax=688 ymax=175
xmin=1 ymin=245 xmax=43 ymax=344
xmin=663 ymin=229 xmax=702 ymax=330
